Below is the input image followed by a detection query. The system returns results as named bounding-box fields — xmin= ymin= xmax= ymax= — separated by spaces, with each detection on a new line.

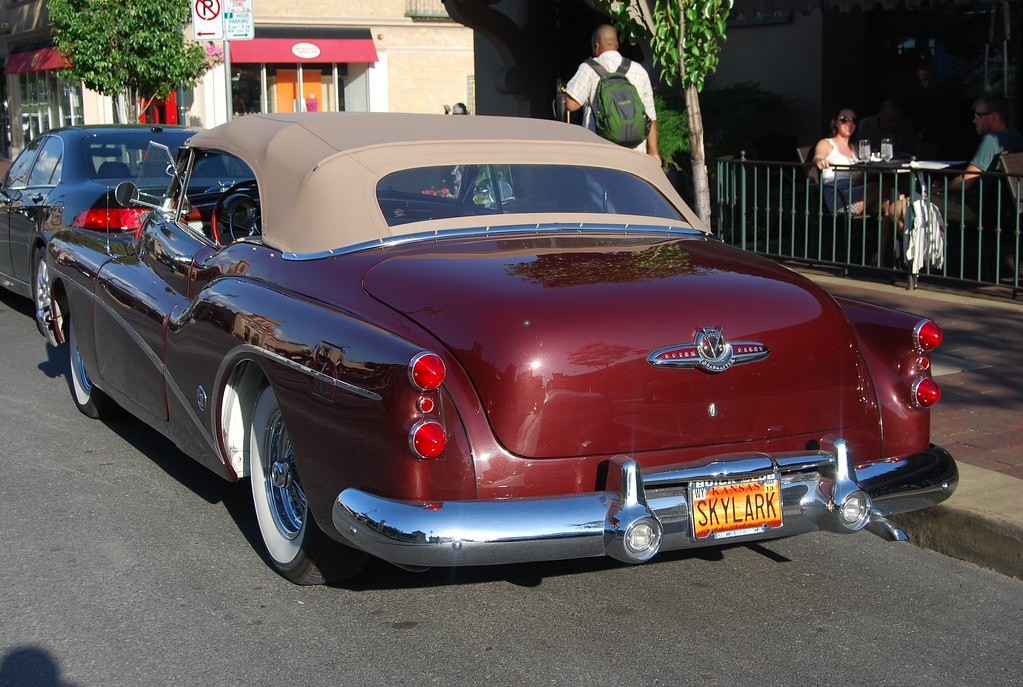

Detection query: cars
xmin=1 ymin=122 xmax=257 ymax=347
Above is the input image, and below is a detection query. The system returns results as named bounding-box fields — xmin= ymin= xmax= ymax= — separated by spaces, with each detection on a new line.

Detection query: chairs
xmin=797 ymin=142 xmax=1023 ymax=283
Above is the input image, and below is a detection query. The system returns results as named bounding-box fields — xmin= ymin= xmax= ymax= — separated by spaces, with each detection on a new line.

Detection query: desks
xmin=850 ymin=156 xmax=957 ymax=272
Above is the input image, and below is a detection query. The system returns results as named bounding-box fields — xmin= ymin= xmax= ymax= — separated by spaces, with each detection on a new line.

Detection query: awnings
xmin=229 ymin=28 xmax=378 ymax=63
xmin=4 ymin=43 xmax=75 ymax=73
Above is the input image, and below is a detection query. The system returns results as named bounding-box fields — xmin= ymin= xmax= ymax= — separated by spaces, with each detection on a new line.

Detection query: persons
xmin=933 ymin=98 xmax=1023 ymax=276
xmin=858 ymin=99 xmax=919 ymax=160
xmin=912 ymin=68 xmax=941 ymax=160
xmin=812 ymin=108 xmax=904 ymax=278
xmin=566 ymin=27 xmax=662 ymax=213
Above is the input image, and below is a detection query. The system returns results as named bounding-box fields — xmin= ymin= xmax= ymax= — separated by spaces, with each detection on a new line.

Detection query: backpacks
xmin=586 ymin=57 xmax=652 ymax=149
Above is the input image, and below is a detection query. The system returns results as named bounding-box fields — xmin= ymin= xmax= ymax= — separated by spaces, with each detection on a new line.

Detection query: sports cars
xmin=44 ymin=104 xmax=960 ymax=586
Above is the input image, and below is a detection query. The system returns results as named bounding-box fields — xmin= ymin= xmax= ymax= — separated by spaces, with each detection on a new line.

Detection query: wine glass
xmin=858 ymin=140 xmax=871 ymax=165
xmin=881 ymin=138 xmax=893 ymax=162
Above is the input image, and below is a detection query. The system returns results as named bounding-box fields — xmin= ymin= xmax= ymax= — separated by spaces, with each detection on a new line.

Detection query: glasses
xmin=841 ymin=116 xmax=857 ymax=124
xmin=975 ymin=112 xmax=992 ymax=119
